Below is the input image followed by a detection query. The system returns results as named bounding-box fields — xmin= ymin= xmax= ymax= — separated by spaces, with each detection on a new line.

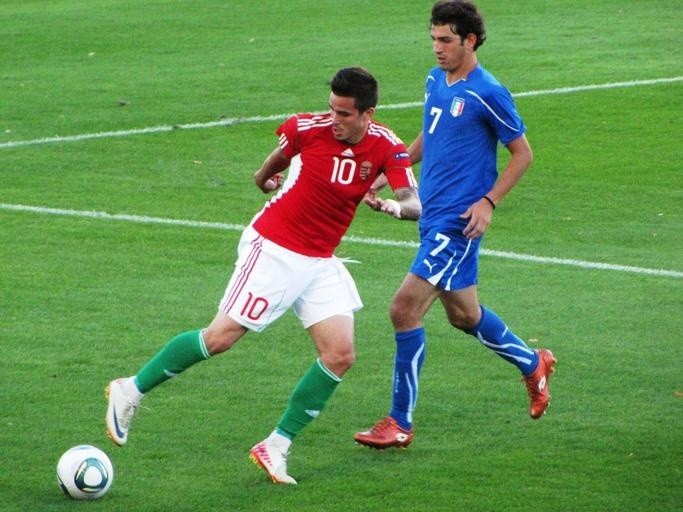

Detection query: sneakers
xmin=248 ymin=440 xmax=297 ymax=485
xmin=353 ymin=416 xmax=414 ymax=451
xmin=105 ymin=377 xmax=150 ymax=448
xmin=522 ymin=347 xmax=557 ymax=419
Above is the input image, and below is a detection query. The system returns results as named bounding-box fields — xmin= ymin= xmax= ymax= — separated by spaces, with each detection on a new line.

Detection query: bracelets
xmin=385 ymin=197 xmax=401 ymax=214
xmin=482 ymin=196 xmax=496 ymax=211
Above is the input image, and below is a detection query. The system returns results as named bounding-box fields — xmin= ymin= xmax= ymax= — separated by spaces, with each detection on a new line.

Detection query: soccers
xmin=56 ymin=444 xmax=113 ymax=502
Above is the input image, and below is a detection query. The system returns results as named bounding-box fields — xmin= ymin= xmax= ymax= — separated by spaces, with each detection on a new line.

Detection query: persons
xmin=103 ymin=66 xmax=423 ymax=486
xmin=352 ymin=2 xmax=556 ymax=450
